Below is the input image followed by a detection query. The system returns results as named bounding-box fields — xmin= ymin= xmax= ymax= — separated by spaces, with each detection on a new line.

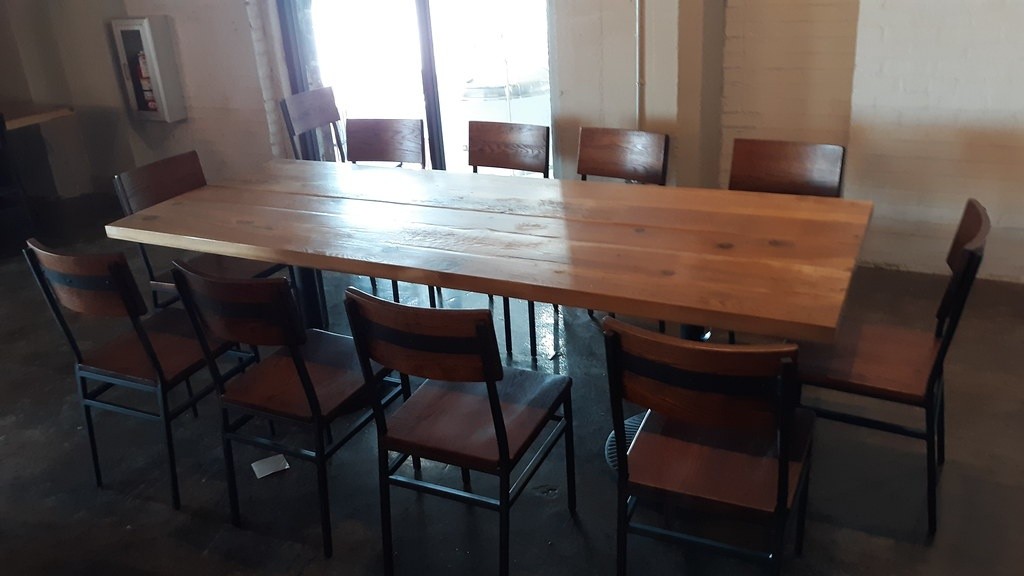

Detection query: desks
xmin=106 ymin=155 xmax=875 ymax=382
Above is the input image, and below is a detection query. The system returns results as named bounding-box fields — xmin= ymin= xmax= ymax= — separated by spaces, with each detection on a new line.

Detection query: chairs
xmin=23 ymin=86 xmax=990 ymax=576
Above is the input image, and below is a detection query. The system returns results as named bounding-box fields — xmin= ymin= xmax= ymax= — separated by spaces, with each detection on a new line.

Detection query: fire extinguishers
xmin=132 ymin=51 xmax=157 ymax=112
